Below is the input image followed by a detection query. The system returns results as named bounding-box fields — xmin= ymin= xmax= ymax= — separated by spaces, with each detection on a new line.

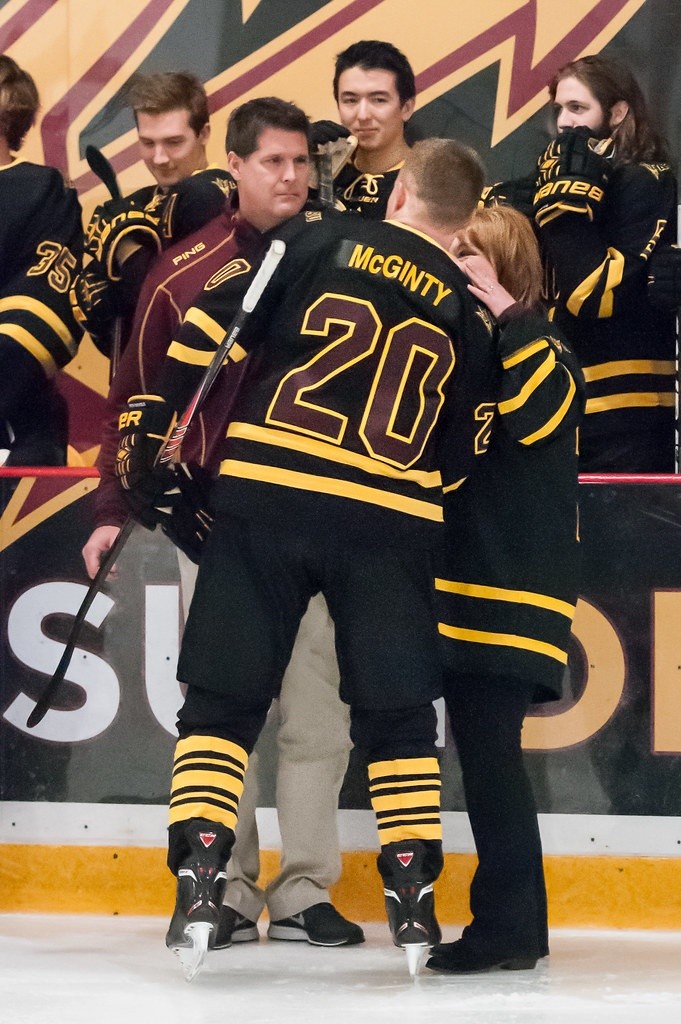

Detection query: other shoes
xmin=423 ymin=917 xmax=545 ymax=975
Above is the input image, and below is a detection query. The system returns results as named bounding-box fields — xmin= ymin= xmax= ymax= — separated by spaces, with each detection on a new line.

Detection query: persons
xmin=0 ymin=53 xmax=86 ymax=469
xmin=486 ymin=55 xmax=681 ymax=475
xmin=69 ymin=40 xmax=587 ymax=975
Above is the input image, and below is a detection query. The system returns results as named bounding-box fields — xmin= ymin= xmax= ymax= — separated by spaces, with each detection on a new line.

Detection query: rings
xmin=486 ymin=285 xmax=494 ymax=295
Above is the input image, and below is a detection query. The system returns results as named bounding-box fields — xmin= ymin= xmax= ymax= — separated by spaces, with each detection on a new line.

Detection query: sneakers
xmin=165 ymin=816 xmax=238 ymax=982
xmin=267 ymin=901 xmax=365 ymax=947
xmin=212 ymin=904 xmax=259 ymax=950
xmin=379 ymin=840 xmax=442 ymax=977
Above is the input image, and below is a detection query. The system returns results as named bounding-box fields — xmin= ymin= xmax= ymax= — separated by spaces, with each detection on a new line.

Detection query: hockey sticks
xmin=25 ymin=239 xmax=286 ymax=728
xmin=84 ymin=145 xmax=123 ymax=380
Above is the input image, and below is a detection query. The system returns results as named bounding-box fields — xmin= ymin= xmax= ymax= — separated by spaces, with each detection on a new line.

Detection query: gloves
xmin=85 ymin=202 xmax=161 ymax=283
xmin=115 ymin=394 xmax=189 ymax=526
xmin=532 ymin=126 xmax=616 ymax=231
